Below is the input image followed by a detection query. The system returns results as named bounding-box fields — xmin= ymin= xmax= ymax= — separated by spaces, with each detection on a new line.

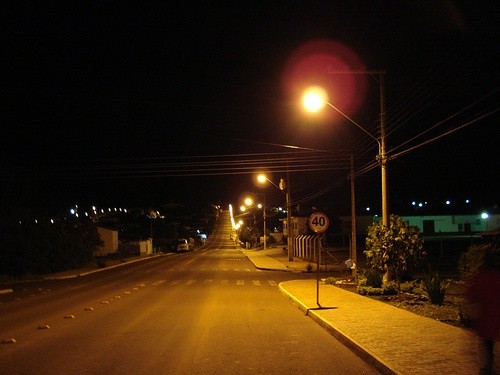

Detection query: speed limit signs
xmin=309 ymin=212 xmax=329 ymax=234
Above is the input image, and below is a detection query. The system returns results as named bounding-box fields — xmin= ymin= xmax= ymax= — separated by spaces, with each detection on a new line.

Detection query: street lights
xmin=240 ymin=206 xmax=257 ymax=248
xmin=257 ymin=174 xmax=293 ymax=261
xmin=244 ymin=198 xmax=266 ymax=251
xmin=305 ymin=91 xmax=387 ymax=289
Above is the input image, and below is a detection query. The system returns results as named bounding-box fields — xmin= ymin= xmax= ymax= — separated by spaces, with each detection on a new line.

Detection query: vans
xmin=176 ymin=239 xmax=188 ymax=252
